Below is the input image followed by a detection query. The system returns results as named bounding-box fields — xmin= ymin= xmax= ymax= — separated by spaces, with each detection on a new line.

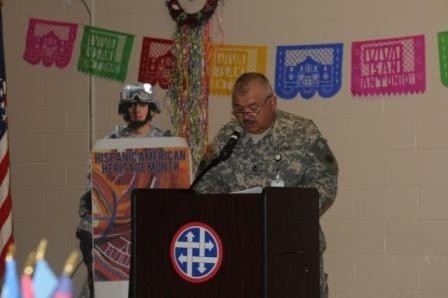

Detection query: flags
xmin=0 ymin=9 xmax=73 ymax=298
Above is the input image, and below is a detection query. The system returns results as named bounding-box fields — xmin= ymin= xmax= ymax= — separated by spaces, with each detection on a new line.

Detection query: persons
xmin=194 ymin=72 xmax=339 ymax=298
xmin=76 ymin=81 xmax=177 ymax=298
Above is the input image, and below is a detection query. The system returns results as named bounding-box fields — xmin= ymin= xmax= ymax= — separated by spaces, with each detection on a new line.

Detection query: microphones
xmin=221 ymin=126 xmax=244 ymax=160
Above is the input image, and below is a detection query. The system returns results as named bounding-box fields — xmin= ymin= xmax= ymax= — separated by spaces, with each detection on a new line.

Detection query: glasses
xmin=121 ymin=83 xmax=153 ymax=100
xmin=230 ymin=94 xmax=272 ymax=118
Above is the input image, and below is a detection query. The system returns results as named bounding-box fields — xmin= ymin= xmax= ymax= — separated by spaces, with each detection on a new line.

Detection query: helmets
xmin=118 ymin=82 xmax=161 ymax=116
